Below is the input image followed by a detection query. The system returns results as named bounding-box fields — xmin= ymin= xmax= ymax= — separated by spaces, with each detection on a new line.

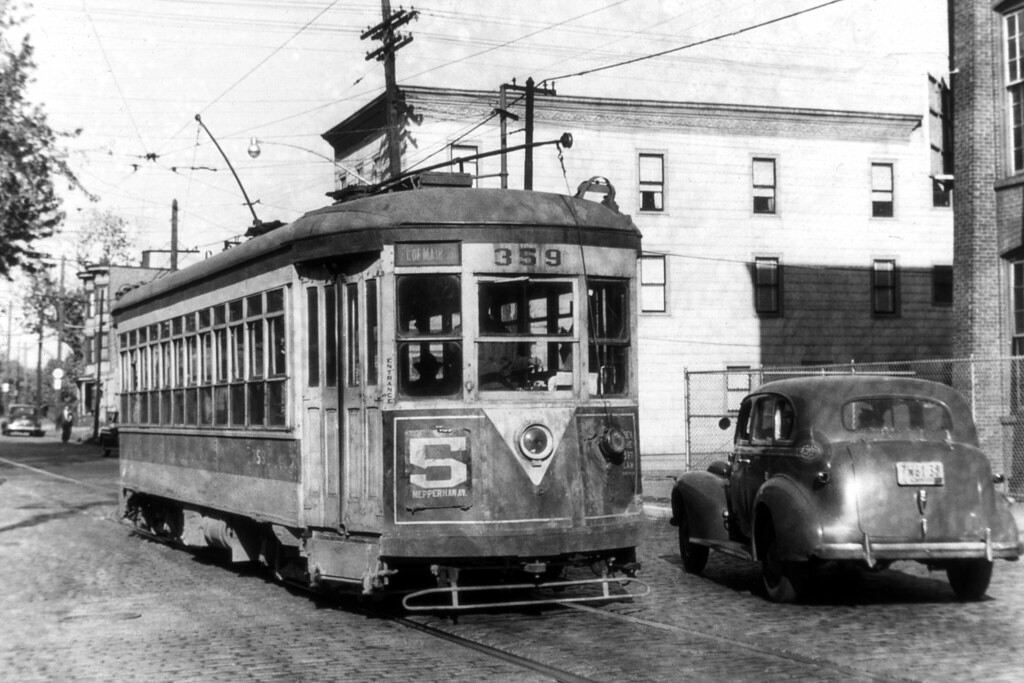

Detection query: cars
xmin=667 ymin=373 xmax=1022 ymax=601
xmin=1 ymin=404 xmax=42 ymax=436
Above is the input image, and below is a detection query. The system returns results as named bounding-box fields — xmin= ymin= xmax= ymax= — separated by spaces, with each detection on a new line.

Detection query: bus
xmin=115 ymin=115 xmax=653 ymax=615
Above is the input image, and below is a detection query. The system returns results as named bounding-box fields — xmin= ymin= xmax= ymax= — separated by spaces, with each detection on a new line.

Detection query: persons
xmin=56 ymin=404 xmax=75 ymax=443
xmin=447 ymin=310 xmax=518 ymax=393
xmin=858 ymin=409 xmax=877 ymax=430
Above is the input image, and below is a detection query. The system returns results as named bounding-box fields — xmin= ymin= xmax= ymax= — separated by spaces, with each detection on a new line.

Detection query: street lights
xmin=87 ymin=274 xmax=111 ymax=448
